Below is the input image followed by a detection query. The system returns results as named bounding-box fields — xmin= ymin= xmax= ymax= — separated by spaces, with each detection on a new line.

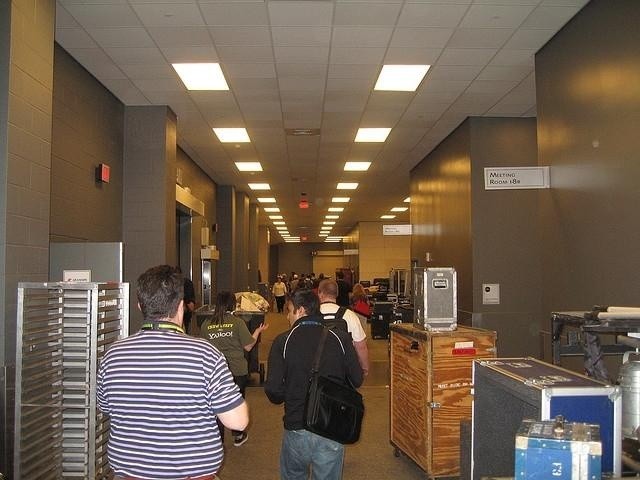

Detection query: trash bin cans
xmin=233 ymin=311 xmax=264 ymax=382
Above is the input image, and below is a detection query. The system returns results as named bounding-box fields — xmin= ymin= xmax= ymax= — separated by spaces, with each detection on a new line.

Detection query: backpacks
xmin=319 ymin=305 xmax=348 ymax=332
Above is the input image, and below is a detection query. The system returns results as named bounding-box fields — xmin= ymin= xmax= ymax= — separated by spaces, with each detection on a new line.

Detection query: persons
xmin=350 ymin=283 xmax=372 ymax=320
xmin=315 ymin=278 xmax=369 ymax=382
xmin=96 ymin=265 xmax=249 ymax=480
xmin=335 ymin=271 xmax=351 ymax=307
xmin=263 ymin=289 xmax=362 ymax=480
xmin=199 ymin=288 xmax=269 ymax=446
xmin=179 ymin=265 xmax=195 ymax=331
xmin=272 ymin=272 xmax=325 ymax=312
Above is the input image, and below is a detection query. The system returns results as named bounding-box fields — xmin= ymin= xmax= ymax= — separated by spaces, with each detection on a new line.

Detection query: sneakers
xmin=233 ymin=433 xmax=247 ymax=446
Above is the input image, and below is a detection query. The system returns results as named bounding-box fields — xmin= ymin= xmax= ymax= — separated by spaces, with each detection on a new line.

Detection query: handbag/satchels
xmin=305 ymin=371 xmax=364 ymax=445
xmin=353 ymin=300 xmax=369 ymax=315
